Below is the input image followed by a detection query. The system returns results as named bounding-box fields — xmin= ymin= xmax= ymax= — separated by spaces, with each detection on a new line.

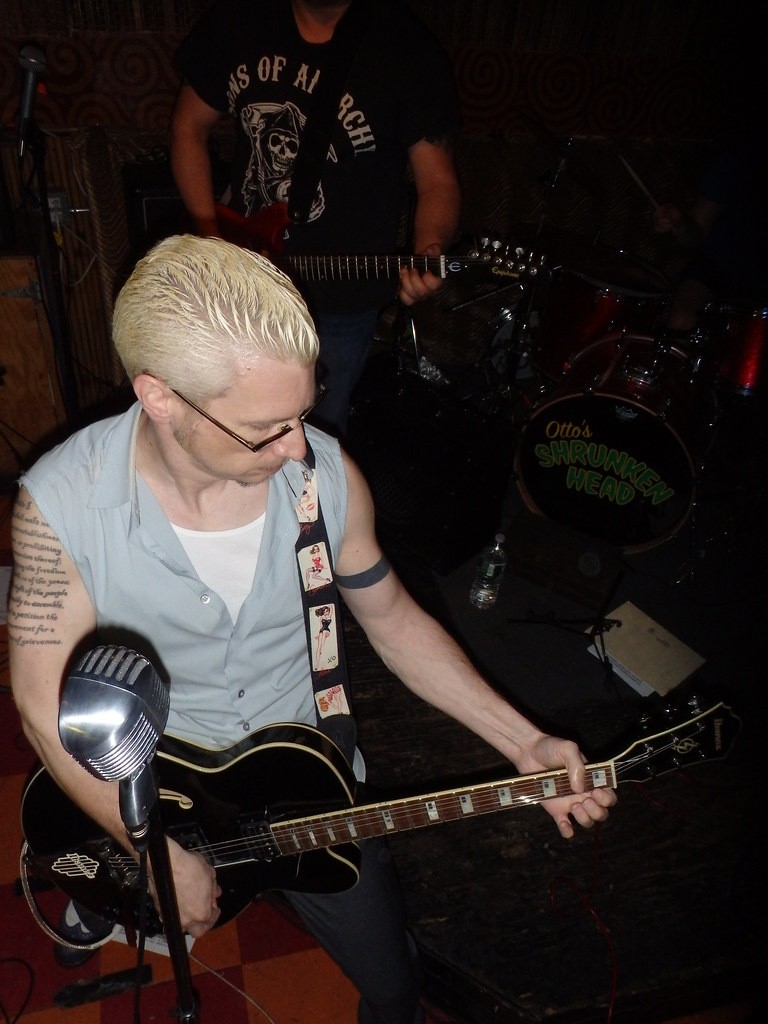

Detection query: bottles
xmin=470 ymin=533 xmax=510 ymax=610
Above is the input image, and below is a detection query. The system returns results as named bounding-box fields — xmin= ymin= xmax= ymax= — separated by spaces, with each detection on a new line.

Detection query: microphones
xmin=58 ymin=645 xmax=171 ymax=852
xmin=16 ymin=46 xmax=47 ymax=169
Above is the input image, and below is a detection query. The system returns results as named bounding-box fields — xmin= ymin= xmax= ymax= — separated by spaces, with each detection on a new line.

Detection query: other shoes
xmin=55 ymin=898 xmax=115 ymax=967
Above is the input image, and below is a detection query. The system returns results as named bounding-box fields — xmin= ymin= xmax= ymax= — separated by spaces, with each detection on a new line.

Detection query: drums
xmin=694 ymin=302 xmax=768 ymax=401
xmin=513 ymin=334 xmax=719 ymax=553
xmin=534 ymin=247 xmax=671 ymax=370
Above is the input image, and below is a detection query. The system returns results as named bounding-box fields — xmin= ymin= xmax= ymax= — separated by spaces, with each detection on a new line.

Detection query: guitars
xmin=19 ymin=693 xmax=745 ymax=949
xmin=159 ymin=201 xmax=538 ymax=283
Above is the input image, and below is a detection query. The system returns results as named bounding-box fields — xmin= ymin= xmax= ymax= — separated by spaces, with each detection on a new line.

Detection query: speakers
xmin=122 ymin=162 xmax=236 ymax=263
xmin=341 ymin=369 xmax=520 ymax=575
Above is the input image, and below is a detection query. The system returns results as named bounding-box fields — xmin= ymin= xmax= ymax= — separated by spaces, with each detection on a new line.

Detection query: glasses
xmin=142 ymin=369 xmax=325 ymax=453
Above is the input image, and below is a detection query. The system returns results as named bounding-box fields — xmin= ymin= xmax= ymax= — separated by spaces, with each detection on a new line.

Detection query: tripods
xmin=505 ymin=564 xmax=634 ymax=720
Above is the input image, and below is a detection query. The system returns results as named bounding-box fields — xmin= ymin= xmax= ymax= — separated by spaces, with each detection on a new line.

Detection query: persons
xmin=5 ymin=233 xmax=616 ymax=1024
xmin=650 ymin=138 xmax=768 ymax=304
xmin=168 ymin=0 xmax=460 ymax=450
